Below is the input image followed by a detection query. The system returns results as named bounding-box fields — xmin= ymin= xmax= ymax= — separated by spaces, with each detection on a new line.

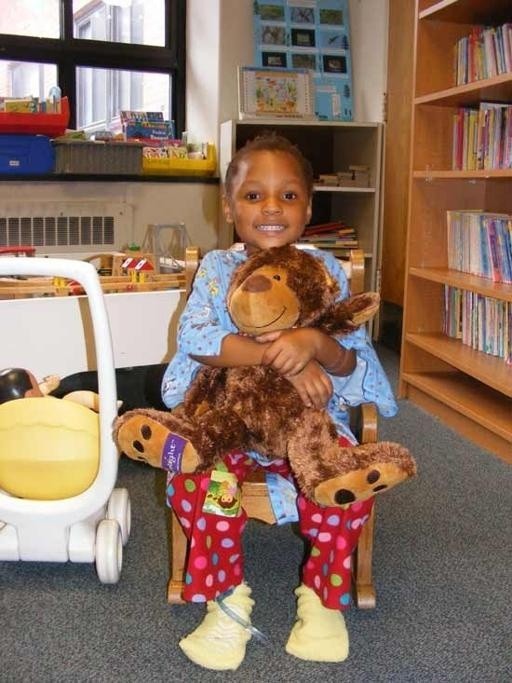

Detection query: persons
xmin=161 ymin=137 xmax=398 ymax=671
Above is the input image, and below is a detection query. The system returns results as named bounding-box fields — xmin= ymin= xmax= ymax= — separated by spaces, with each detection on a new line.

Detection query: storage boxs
xmin=48 ymin=139 xmax=146 ymax=177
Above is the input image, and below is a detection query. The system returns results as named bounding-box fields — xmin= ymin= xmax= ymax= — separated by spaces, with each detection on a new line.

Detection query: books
xmin=444 ymin=22 xmax=512 ymax=363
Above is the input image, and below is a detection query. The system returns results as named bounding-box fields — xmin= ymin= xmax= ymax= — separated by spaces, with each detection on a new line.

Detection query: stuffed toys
xmin=111 ymin=246 xmax=417 ymax=511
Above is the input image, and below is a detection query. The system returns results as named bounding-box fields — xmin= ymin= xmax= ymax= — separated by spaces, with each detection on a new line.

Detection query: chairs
xmin=163 ymin=245 xmax=380 ymax=609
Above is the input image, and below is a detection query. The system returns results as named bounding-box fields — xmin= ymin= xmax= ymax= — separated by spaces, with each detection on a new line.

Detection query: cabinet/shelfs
xmin=217 ymin=119 xmax=385 ymax=339
xmin=397 ymin=0 xmax=512 ymax=468
